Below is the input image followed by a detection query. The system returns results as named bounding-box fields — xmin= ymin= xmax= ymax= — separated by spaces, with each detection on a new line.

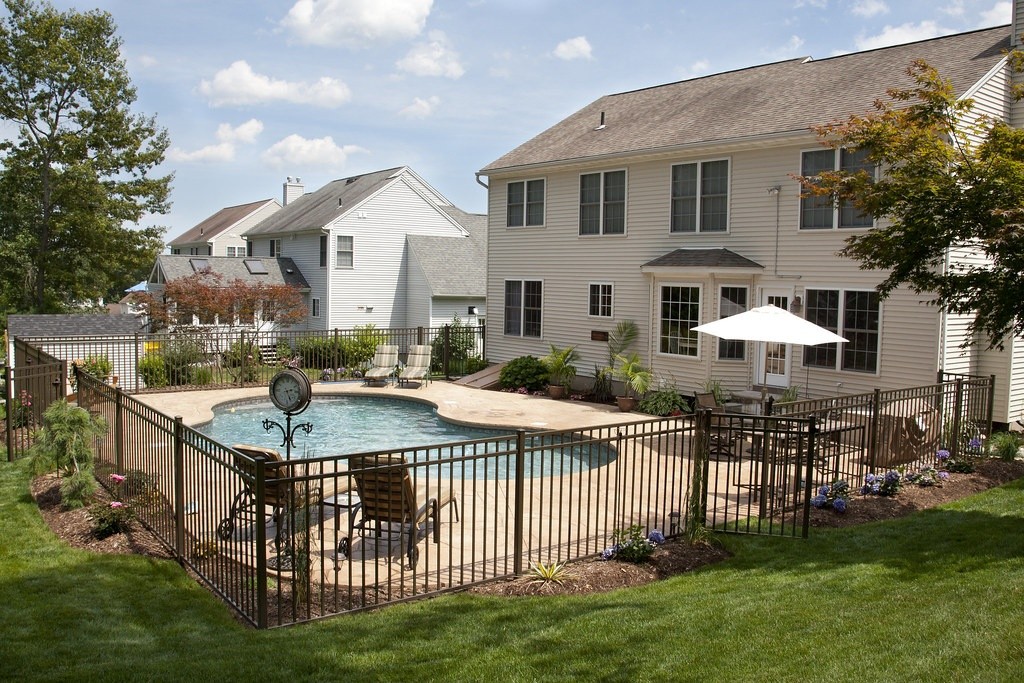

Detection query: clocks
xmin=262 ymin=365 xmax=313 ymax=570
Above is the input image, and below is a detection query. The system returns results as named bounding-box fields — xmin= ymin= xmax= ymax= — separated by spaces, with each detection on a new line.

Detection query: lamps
xmin=778 ymin=484 xmax=784 ymax=500
xmin=790 ymin=297 xmax=802 ymax=313
xmin=768 ymin=186 xmax=780 ymax=196
xmin=800 ymin=479 xmax=805 ymax=491
xmin=330 ymin=553 xmax=346 ymax=572
xmin=668 ymin=511 xmax=682 ymax=526
xmin=469 ymin=304 xmax=479 ymax=316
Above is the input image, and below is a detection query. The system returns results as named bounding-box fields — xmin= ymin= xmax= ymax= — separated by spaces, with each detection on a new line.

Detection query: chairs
xmin=669 ymin=331 xmax=684 ymax=354
xmin=399 ymin=344 xmax=433 ymax=389
xmin=335 ymin=453 xmax=458 ymax=570
xmin=693 ymin=389 xmax=759 ymax=457
xmin=218 ymin=444 xmax=356 ymax=546
xmin=364 ymin=344 xmax=399 ymax=387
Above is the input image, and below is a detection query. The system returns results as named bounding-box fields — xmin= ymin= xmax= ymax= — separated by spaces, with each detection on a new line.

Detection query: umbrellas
xmin=690 ymin=303 xmax=851 ymax=400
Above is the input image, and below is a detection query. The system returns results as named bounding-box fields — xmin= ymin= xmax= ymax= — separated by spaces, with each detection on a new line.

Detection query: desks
xmin=679 ymin=340 xmax=698 ymax=348
xmin=317 ymin=494 xmax=362 ymax=541
xmin=736 ymin=417 xmax=864 ymax=501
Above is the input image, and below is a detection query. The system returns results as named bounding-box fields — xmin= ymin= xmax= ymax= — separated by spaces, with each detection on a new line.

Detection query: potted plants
xmin=599 ymin=352 xmax=651 ymax=413
xmin=539 ymin=341 xmax=581 ymax=399
xmin=634 ymin=369 xmax=690 ymax=417
xmin=775 ymin=383 xmax=803 ymax=413
